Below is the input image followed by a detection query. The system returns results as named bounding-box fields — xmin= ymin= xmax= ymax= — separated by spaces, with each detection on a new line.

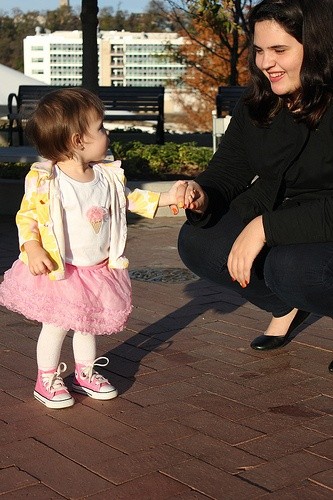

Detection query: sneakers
xmin=71 ymin=357 xmax=118 ymax=401
xmin=33 ymin=362 xmax=75 ymax=409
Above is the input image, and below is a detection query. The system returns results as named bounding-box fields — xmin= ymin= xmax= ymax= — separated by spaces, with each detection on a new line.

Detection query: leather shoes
xmin=250 ymin=308 xmax=312 ymax=351
xmin=328 ymin=360 xmax=333 ymax=373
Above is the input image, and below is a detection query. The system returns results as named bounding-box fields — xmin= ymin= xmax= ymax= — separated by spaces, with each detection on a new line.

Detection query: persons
xmin=169 ymin=0 xmax=333 ymax=376
xmin=0 ymin=88 xmax=195 ymax=410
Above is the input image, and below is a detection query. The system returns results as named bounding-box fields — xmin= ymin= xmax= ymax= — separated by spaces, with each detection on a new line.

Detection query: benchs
xmin=6 ymin=85 xmax=165 ymax=147
xmin=216 ymin=85 xmax=253 ymax=117
